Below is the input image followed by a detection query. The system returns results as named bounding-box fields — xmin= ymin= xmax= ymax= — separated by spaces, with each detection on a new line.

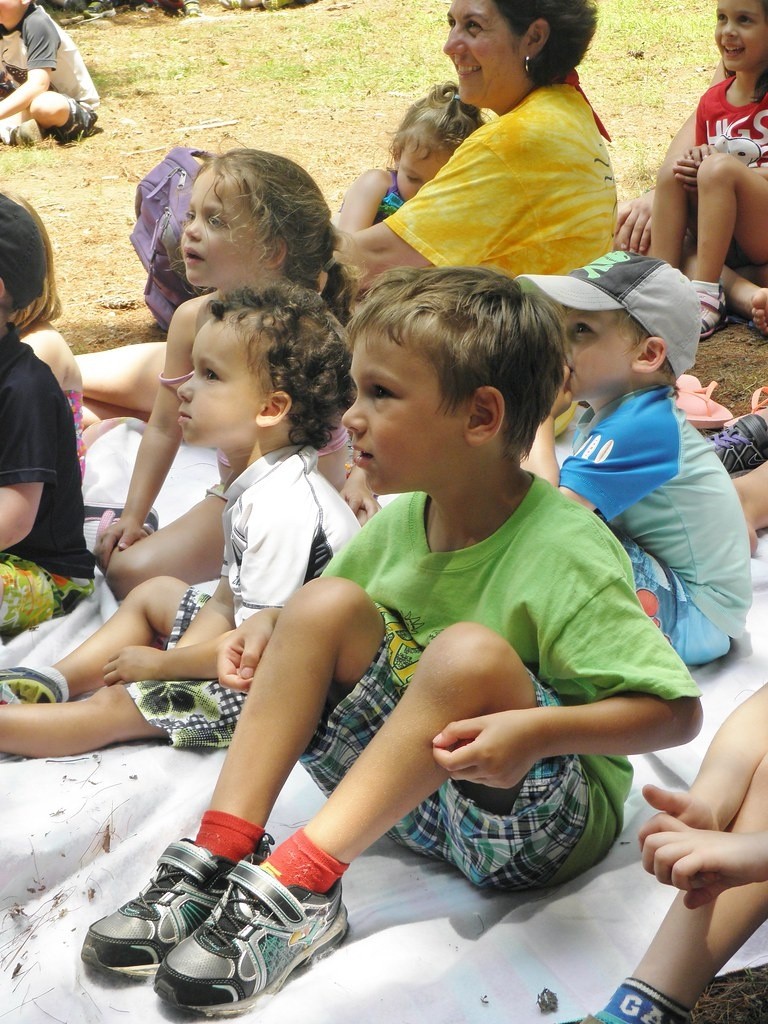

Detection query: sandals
xmin=696 ymin=280 xmax=728 ymax=339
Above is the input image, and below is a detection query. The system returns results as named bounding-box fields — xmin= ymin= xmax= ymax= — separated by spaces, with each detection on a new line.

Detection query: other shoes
xmin=83 ymin=499 xmax=159 ymax=541
xmin=13 ymin=119 xmax=43 ymax=146
xmin=0 ymin=667 xmax=62 ymax=704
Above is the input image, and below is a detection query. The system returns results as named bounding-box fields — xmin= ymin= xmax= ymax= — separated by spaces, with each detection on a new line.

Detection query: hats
xmin=513 ymin=250 xmax=701 ymax=380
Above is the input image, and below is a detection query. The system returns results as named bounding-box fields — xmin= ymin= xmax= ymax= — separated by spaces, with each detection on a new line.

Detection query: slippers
xmin=674 ymin=374 xmax=734 ymax=430
xmin=724 ymin=387 xmax=768 ymax=428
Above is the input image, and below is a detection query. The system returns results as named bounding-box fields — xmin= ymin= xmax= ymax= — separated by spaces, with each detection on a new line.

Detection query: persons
xmin=80 ymin=263 xmax=710 ymax=1017
xmin=553 ymin=680 xmax=768 ymax=1024
xmin=94 ymin=150 xmax=390 ymax=611
xmin=333 ymin=0 xmax=622 ymax=303
xmin=508 ymin=251 xmax=751 ymax=670
xmin=612 ymin=0 xmax=768 ymax=431
xmin=1 ymin=284 xmax=372 ymax=762
xmin=1 ymin=194 xmax=100 ymax=629
xmin=0 ymin=0 xmax=100 ymax=146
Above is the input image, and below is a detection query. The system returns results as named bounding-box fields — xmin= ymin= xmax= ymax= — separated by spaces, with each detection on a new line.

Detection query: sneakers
xmin=183 ymin=0 xmax=202 ymax=18
xmin=81 ymin=833 xmax=275 ymax=981
xmin=84 ymin=0 xmax=116 ymax=19
xmin=704 ymin=413 xmax=768 ymax=478
xmin=154 ymin=860 xmax=349 ymax=1018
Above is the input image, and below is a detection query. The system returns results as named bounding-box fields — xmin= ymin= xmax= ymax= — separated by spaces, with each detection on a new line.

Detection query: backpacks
xmin=129 ymin=146 xmax=217 ymax=332
